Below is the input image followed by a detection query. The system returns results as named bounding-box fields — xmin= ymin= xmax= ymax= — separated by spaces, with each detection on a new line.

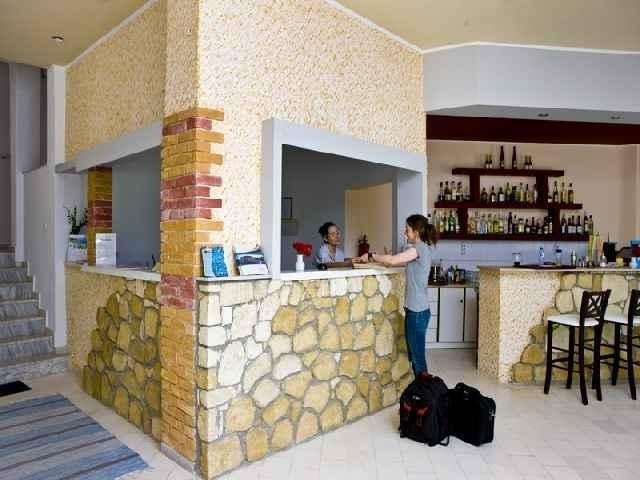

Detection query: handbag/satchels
xmin=448 ymin=384 xmax=495 ymax=446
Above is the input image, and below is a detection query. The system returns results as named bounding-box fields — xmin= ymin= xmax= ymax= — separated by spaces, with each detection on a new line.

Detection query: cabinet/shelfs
xmin=425 ymin=280 xmax=478 ymax=349
xmin=428 ymin=167 xmax=592 ymax=242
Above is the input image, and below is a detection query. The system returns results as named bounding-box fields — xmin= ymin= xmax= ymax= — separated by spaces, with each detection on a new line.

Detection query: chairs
xmin=544 ymin=289 xmax=612 ymax=405
xmin=592 ymin=289 xmax=640 ymax=400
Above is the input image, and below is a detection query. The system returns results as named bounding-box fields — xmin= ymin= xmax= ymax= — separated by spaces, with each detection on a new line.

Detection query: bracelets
xmin=368 ymin=251 xmax=373 ymax=261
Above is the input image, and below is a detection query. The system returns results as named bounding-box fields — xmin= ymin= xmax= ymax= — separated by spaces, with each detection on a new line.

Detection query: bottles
xmin=511 ymin=145 xmax=517 ymax=168
xmin=505 ymin=181 xmax=512 ymax=203
xmin=481 ymin=187 xmax=488 ymax=203
xmin=568 ymin=183 xmax=575 ymax=204
xmin=523 ymin=154 xmax=529 ymax=170
xmin=468 ymin=210 xmax=553 ymax=234
xmin=457 ymin=181 xmax=463 ymax=201
xmin=497 ymin=186 xmax=505 ymax=203
xmin=553 ymin=180 xmax=559 ymax=203
xmin=489 ymin=185 xmax=497 ymax=203
xmin=510 ymin=185 xmax=519 ymax=203
xmin=485 ymin=153 xmax=490 ymax=169
xmin=526 ymin=183 xmax=531 ymax=203
xmin=489 ymin=154 xmax=494 ymax=169
xmin=431 ymin=257 xmax=466 ymax=284
xmin=438 ymin=181 xmax=444 ymax=200
xmin=499 ymin=145 xmax=505 ymax=169
xmin=561 ymin=211 xmax=594 ymax=235
xmin=532 ymin=184 xmax=538 ymax=203
xmin=537 ymin=245 xmax=545 ymax=267
xmin=444 ymin=180 xmax=451 ymax=200
xmin=295 ymin=254 xmax=305 ymax=272
xmin=427 ymin=209 xmax=461 ymax=234
xmin=554 ymin=244 xmax=563 ymax=266
xmin=559 ymin=182 xmax=567 ymax=204
xmin=451 ymin=180 xmax=456 ymax=200
xmin=529 ymin=154 xmax=533 ymax=170
xmin=518 ymin=182 xmax=526 ymax=203
xmin=464 ymin=186 xmax=470 ymax=201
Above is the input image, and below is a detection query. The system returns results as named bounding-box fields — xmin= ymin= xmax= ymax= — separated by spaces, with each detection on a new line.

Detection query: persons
xmin=364 ymin=214 xmax=438 ymax=379
xmin=313 ymin=221 xmax=362 ymax=268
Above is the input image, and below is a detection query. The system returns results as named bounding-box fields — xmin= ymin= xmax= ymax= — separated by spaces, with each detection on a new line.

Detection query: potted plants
xmin=65 ymin=207 xmax=88 ymax=248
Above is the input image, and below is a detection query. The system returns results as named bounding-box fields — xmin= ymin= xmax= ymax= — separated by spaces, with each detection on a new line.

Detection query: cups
xmin=368 ymin=251 xmax=377 ymax=263
xmin=512 ymin=252 xmax=523 ymax=268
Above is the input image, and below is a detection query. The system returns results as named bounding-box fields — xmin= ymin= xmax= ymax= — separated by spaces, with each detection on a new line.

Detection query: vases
xmin=296 ymin=255 xmax=304 ymax=272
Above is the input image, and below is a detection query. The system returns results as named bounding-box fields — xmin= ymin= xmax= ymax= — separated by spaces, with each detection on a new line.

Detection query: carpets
xmin=0 ymin=393 xmax=149 ymax=480
xmin=1 ymin=381 xmax=32 ymax=398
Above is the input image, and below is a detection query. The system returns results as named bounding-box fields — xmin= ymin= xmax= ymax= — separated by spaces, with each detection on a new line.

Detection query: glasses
xmin=327 ymin=231 xmax=340 ymax=236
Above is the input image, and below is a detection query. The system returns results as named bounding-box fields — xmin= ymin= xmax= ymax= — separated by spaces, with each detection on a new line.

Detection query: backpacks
xmin=398 ymin=375 xmax=448 ymax=446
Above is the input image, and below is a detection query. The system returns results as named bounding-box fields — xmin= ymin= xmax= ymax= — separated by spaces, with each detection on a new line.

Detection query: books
xmin=233 ymin=240 xmax=269 ymax=276
xmin=202 ymin=246 xmax=227 ymax=278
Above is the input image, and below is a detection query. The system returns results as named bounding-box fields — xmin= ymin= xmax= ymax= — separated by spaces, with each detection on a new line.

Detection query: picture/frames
xmin=281 ymin=193 xmax=296 ymax=219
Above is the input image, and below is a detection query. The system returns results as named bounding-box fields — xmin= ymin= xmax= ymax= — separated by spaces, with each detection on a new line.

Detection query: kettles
xmin=454 ymin=268 xmax=467 ymax=285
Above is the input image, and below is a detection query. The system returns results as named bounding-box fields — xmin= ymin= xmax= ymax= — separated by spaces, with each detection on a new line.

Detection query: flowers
xmin=293 ymin=241 xmax=312 ymax=256
